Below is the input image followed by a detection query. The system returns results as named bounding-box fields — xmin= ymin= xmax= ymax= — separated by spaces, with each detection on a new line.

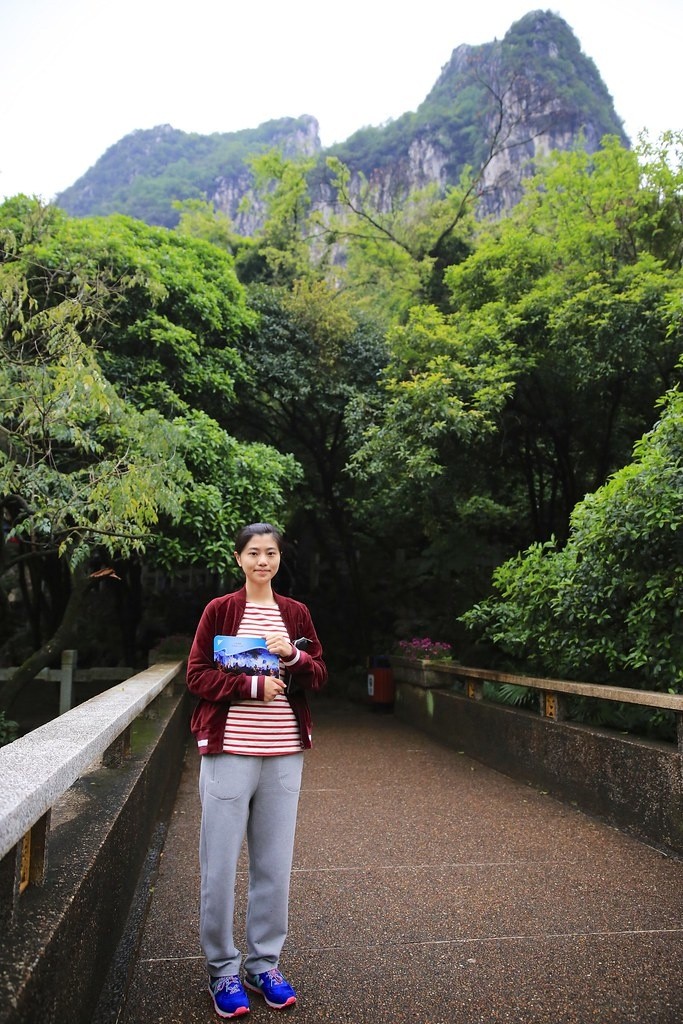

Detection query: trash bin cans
xmin=368 ymin=656 xmax=395 ymax=712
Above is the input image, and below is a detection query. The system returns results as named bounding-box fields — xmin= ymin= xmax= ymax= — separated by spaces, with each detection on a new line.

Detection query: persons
xmin=185 ymin=521 xmax=325 ymax=1018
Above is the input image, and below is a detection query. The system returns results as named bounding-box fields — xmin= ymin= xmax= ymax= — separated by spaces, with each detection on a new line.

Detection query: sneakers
xmin=208 ymin=974 xmax=250 ymax=1018
xmin=244 ymin=969 xmax=296 ymax=1009
